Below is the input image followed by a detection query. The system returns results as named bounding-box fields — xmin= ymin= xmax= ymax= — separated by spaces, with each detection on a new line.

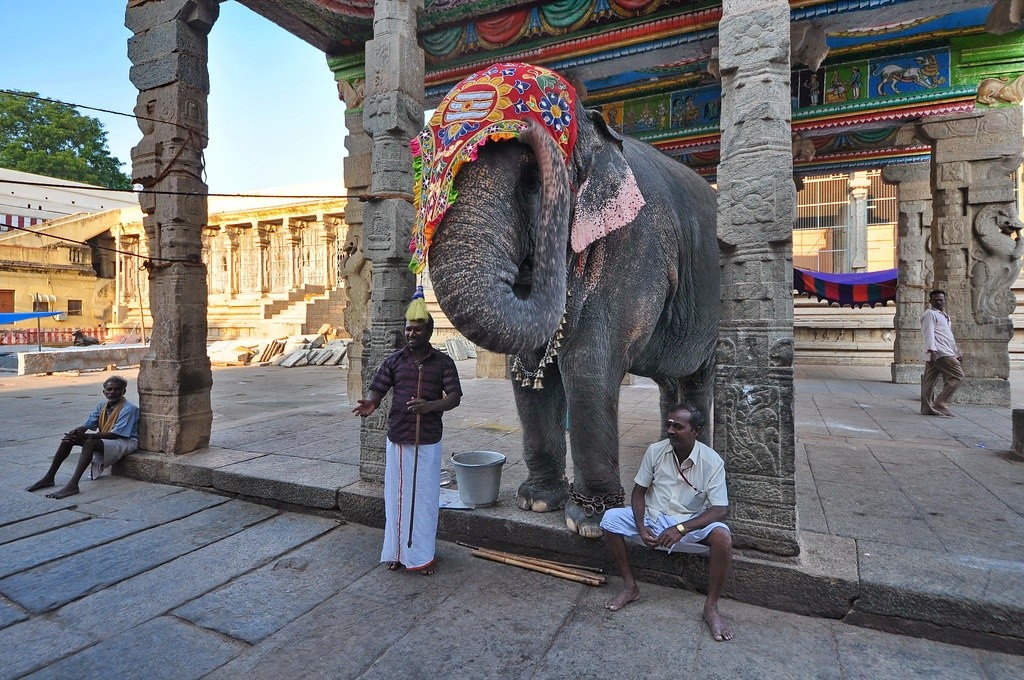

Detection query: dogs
xmin=72 ymin=330 xmax=99 ymax=347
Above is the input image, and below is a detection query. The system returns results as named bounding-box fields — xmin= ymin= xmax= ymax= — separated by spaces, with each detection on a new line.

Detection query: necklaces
xmin=671 ymin=452 xmax=697 ymax=493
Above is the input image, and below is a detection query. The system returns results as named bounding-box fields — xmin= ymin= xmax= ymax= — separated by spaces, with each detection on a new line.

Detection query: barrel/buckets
xmin=450 ymin=449 xmax=507 ymax=508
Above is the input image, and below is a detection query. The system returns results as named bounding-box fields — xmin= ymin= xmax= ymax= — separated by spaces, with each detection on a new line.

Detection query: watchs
xmin=677 ymin=524 xmax=686 ymax=537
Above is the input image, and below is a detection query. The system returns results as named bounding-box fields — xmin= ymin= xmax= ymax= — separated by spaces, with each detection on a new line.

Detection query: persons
xmin=594 ymin=403 xmax=736 ymax=642
xmin=24 ymin=375 xmax=139 ymax=500
xmin=920 ymin=288 xmax=963 ymax=416
xmin=351 ymin=310 xmax=463 ymax=575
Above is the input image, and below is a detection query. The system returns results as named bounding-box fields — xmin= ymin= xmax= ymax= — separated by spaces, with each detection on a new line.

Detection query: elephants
xmin=409 ymin=64 xmax=721 ymax=539
